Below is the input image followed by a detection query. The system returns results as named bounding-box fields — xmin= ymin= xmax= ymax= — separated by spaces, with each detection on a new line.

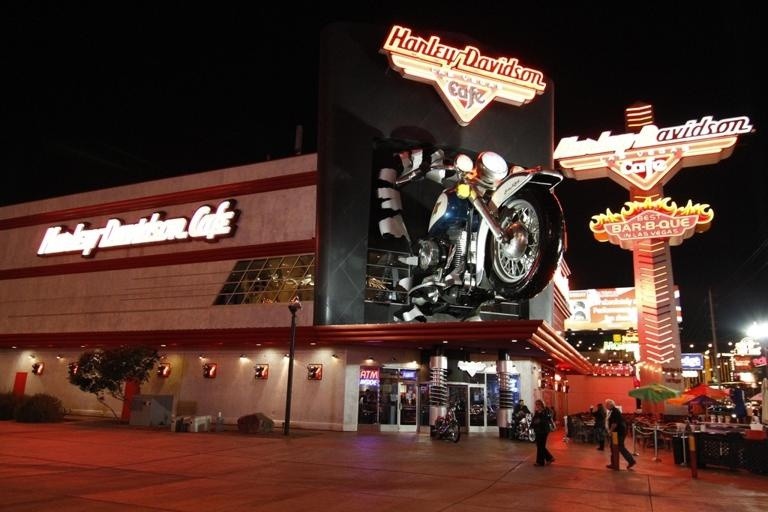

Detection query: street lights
xmin=283 ymin=295 xmax=302 ymax=436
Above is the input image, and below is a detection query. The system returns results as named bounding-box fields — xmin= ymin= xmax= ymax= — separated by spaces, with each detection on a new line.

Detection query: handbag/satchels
xmin=612 ymin=431 xmax=618 ymax=449
xmin=548 ymin=414 xmax=556 ymax=432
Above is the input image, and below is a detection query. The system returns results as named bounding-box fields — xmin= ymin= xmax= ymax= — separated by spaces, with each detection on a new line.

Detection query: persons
xmin=509 ymin=400 xmax=530 ymax=440
xmin=589 ymin=403 xmax=607 ymax=451
xmin=604 ymin=399 xmax=636 ymax=469
xmin=526 ymin=399 xmax=556 ymax=467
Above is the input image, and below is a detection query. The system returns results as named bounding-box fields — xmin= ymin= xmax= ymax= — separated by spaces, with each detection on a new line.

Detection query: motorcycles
xmin=374 ymin=141 xmax=566 ymax=324
xmin=507 ymin=411 xmax=536 ymax=442
xmin=433 ymin=400 xmax=460 ymax=443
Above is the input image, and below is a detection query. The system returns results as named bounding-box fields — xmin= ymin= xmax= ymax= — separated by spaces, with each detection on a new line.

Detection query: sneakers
xmin=597 ymin=447 xmax=636 ymax=470
xmin=534 ymin=456 xmax=556 ymax=467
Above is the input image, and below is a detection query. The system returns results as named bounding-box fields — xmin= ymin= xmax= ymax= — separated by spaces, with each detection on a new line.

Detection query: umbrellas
xmin=761 ymin=378 xmax=768 ymax=425
xmin=628 ymin=383 xmax=728 ymax=422
